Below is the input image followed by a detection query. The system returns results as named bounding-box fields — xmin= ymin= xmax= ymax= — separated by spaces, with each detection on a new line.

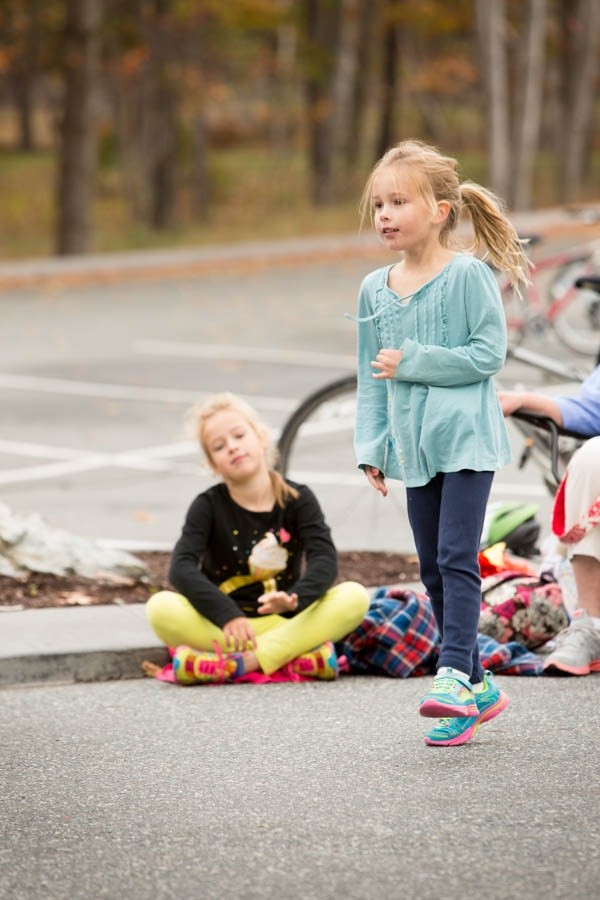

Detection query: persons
xmin=144 ymin=394 xmax=370 ymax=684
xmin=350 ymin=143 xmax=534 ymax=743
xmin=496 ymin=363 xmax=600 ymax=677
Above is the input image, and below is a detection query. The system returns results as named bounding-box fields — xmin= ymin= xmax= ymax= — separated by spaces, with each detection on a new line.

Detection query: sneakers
xmin=420 ymin=667 xmax=479 ymax=717
xmin=294 ymin=643 xmax=339 ymax=681
xmin=425 ymin=675 xmax=509 ymax=746
xmin=173 ymin=645 xmax=245 ymax=686
xmin=542 ymin=609 xmax=600 ymax=676
